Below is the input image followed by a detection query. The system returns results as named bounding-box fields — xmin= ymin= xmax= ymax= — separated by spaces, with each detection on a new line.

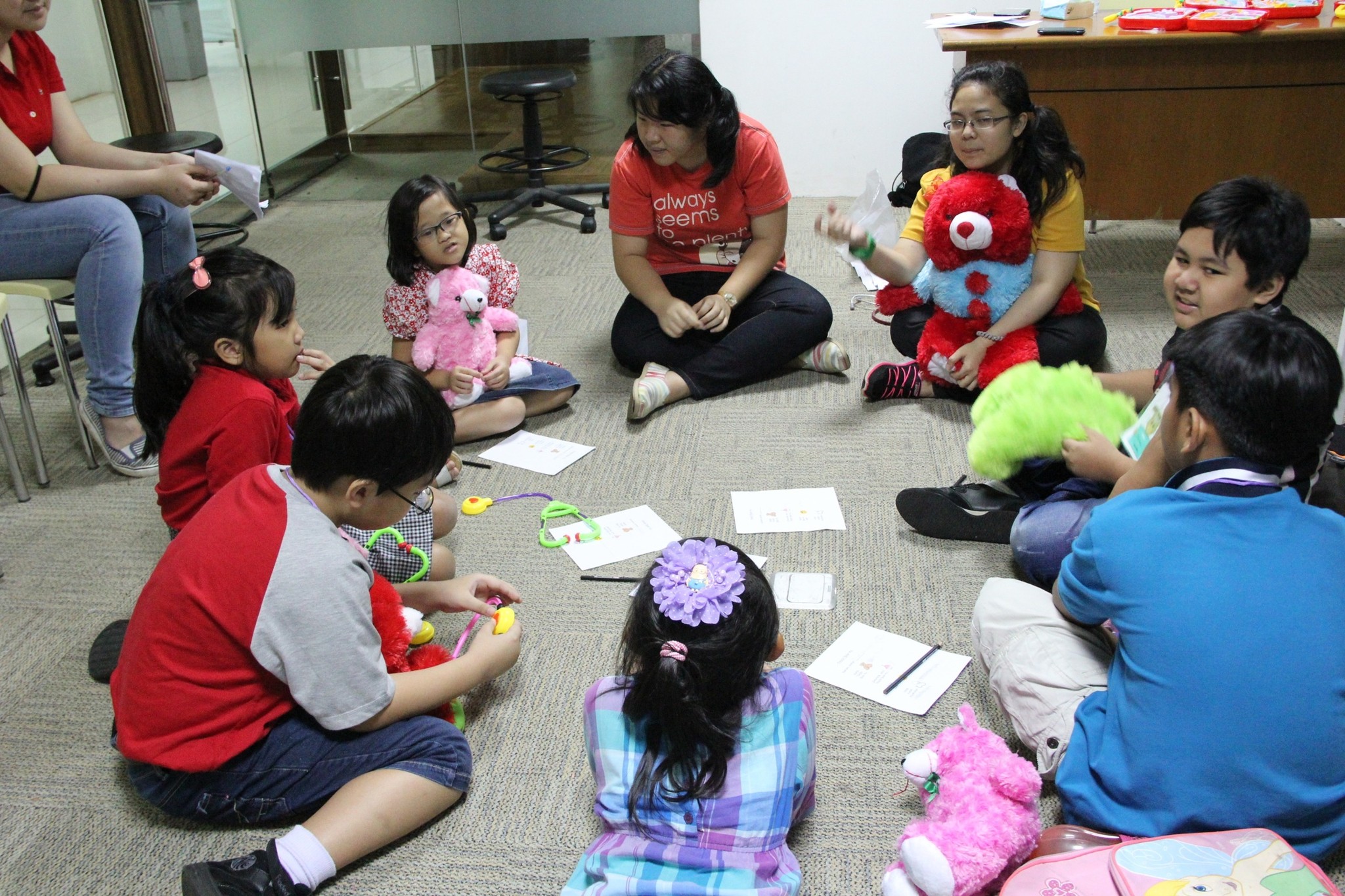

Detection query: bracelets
xmin=22 ymin=163 xmax=42 ymax=202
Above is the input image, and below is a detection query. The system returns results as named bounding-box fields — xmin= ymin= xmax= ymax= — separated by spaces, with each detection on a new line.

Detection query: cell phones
xmin=1037 ymin=27 xmax=1085 ymax=35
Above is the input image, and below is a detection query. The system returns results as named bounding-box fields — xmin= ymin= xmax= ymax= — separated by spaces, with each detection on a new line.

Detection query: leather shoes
xmin=896 ymin=482 xmax=1028 ymax=545
xmin=182 ymin=849 xmax=296 ymax=896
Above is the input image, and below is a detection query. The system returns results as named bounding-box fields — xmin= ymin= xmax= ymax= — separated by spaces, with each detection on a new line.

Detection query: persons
xmin=608 ymin=48 xmax=852 ymax=421
xmin=563 ymin=536 xmax=820 ymax=896
xmin=896 ymin=177 xmax=1312 ymax=589
xmin=1 ymin=0 xmax=225 ymax=480
xmin=87 ymin=353 xmax=526 ymax=896
xmin=133 ymin=247 xmax=461 ymax=580
xmin=973 ymin=309 xmax=1345 ymax=862
xmin=819 ymin=61 xmax=1106 ymax=406
xmin=381 ymin=171 xmax=581 ymax=446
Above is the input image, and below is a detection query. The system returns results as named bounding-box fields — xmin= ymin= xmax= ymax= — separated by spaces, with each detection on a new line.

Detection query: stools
xmin=446 ymin=63 xmax=612 ymax=240
xmin=29 ymin=129 xmax=259 ymax=387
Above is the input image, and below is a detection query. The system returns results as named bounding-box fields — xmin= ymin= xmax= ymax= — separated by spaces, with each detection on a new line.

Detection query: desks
xmin=928 ymin=1 xmax=1343 ymax=221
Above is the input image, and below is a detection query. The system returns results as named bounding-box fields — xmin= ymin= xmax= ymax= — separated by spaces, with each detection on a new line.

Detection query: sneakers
xmin=798 ymin=336 xmax=851 ymax=373
xmin=627 ymin=361 xmax=670 ymax=420
xmin=860 ymin=357 xmax=921 ymax=400
xmin=79 ymin=394 xmax=161 ymax=477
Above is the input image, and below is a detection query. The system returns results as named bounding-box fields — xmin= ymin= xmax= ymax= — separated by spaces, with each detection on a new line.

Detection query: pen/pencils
xmin=580 ymin=575 xmax=641 ymax=583
xmin=883 ymin=642 xmax=944 ymax=694
xmin=462 ymin=461 xmax=491 ymax=470
xmin=202 ymin=166 xmax=230 ymax=181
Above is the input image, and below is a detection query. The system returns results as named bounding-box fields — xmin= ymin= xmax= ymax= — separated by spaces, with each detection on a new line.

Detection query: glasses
xmin=943 ymin=112 xmax=1018 ymax=132
xmin=379 ymin=480 xmax=434 ymax=516
xmin=412 ymin=211 xmax=463 ymax=243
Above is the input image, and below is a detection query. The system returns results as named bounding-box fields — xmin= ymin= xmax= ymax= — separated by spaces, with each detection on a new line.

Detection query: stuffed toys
xmin=966 ymin=359 xmax=1137 ymax=479
xmin=879 ymin=702 xmax=1042 ymax=896
xmin=876 ymin=171 xmax=1084 ymax=390
xmin=411 ymin=266 xmax=517 ymax=409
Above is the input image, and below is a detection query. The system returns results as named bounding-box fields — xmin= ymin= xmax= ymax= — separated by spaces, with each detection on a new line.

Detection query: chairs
xmin=0 ymin=291 xmax=50 ymax=506
xmin=0 ymin=272 xmax=101 ymax=470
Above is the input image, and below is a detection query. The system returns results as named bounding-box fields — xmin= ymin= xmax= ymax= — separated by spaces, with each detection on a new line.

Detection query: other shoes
xmin=88 ymin=619 xmax=131 ymax=684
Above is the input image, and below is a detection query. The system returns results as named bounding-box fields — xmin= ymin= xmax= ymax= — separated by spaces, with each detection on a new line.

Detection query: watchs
xmin=715 ymin=288 xmax=739 ymax=309
xmin=849 ymin=233 xmax=876 ymax=260
